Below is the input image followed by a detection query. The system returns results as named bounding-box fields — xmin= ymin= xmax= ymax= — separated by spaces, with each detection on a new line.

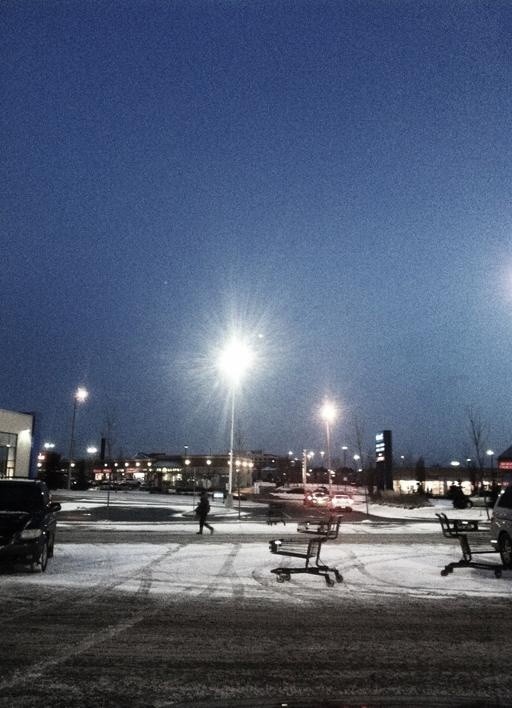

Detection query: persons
xmin=195 ymin=489 xmax=213 ymax=534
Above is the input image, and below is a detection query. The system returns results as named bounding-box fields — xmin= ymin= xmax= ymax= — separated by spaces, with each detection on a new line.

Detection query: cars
xmin=270 ymin=487 xmax=354 ymax=511
xmin=492 ymin=487 xmax=512 ymax=567
xmin=40 ymin=471 xmax=227 ymax=491
xmin=0 ymin=476 xmax=62 ymax=571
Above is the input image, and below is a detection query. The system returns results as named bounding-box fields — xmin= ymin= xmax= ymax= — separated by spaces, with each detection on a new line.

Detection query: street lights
xmin=218 ymin=347 xmax=243 ymax=507
xmin=67 ymin=385 xmax=88 ymax=490
xmin=288 ymin=447 xmax=359 ymax=483
xmin=321 ymin=402 xmax=337 ymax=496
